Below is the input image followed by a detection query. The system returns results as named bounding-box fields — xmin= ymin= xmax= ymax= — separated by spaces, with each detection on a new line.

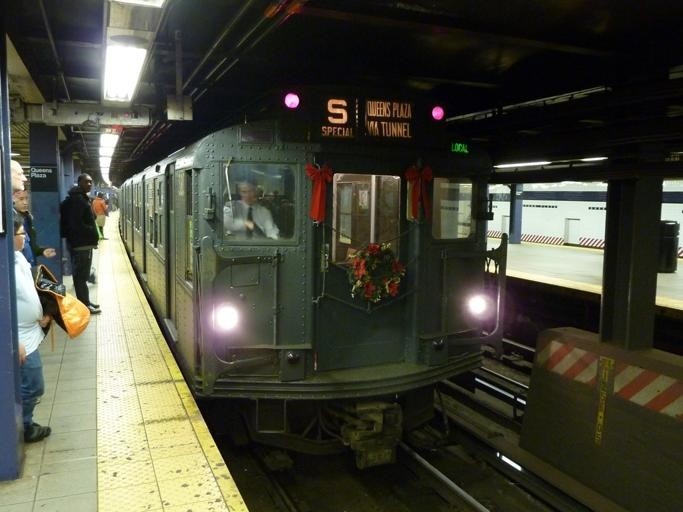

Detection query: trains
xmin=438 ymin=173 xmax=683 ymax=259
xmin=117 ymin=90 xmax=505 ymax=478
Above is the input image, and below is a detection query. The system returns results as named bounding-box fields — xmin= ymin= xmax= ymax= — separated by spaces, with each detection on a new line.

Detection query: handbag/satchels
xmin=32 ymin=264 xmax=90 ymax=339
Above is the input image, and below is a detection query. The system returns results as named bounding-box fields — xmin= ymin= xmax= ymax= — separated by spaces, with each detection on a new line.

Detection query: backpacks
xmin=60 ymin=196 xmax=71 ymax=239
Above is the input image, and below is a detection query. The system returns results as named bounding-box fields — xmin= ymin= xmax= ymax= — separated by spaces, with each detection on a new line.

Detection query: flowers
xmin=346 ymin=242 xmax=407 ymax=305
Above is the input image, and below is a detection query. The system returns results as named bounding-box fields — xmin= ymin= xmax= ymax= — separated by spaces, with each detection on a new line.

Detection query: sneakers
xmin=87 ymin=302 xmax=101 ymax=314
xmin=25 ymin=421 xmax=51 ymax=443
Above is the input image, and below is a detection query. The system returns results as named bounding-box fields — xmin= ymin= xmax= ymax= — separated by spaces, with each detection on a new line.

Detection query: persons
xmin=10 ymin=157 xmax=120 ymax=446
xmin=225 ymin=173 xmax=281 ymax=239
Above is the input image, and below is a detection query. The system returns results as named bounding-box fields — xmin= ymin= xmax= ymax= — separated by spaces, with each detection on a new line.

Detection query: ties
xmin=247 ymin=207 xmax=253 ymax=239
